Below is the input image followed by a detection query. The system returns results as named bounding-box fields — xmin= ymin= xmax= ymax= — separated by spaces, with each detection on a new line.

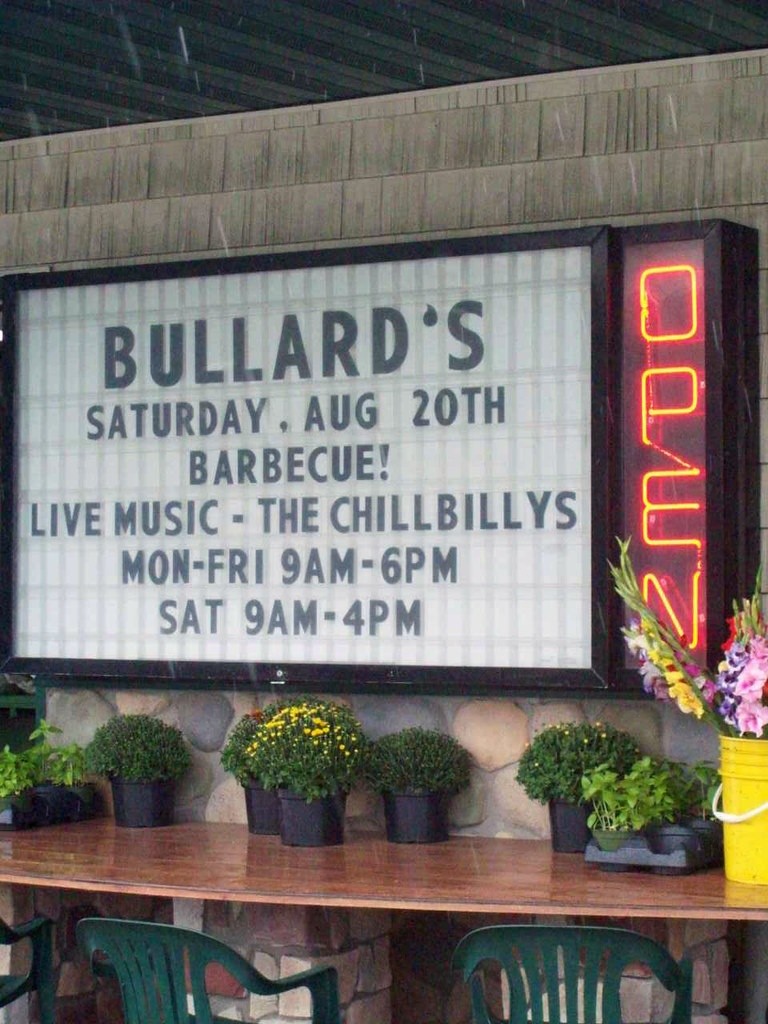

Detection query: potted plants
xmin=219 ymin=696 xmax=326 ymax=836
xmin=581 ymin=756 xmax=721 ymax=876
xmin=0 ymin=719 xmax=100 ymax=830
xmin=363 ymin=726 xmax=474 ymax=843
xmin=83 ymin=713 xmax=194 ymax=827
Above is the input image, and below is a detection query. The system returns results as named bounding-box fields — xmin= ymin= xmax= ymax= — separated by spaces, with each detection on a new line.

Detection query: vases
xmin=548 ymin=801 xmax=597 ymax=852
xmin=274 ymin=785 xmax=347 ymax=846
xmin=711 ymin=734 xmax=768 ymax=884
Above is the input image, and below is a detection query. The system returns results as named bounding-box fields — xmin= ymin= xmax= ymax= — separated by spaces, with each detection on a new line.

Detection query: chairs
xmin=76 ymin=918 xmax=340 ymax=1024
xmin=0 ymin=915 xmax=58 ymax=1024
xmin=446 ymin=925 xmax=692 ymax=1024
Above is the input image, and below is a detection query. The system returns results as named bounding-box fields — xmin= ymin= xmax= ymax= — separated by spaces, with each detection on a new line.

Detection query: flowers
xmin=514 ymin=719 xmax=642 ymax=805
xmin=605 ymin=534 xmax=768 ymax=739
xmin=243 ymin=704 xmax=374 ymax=803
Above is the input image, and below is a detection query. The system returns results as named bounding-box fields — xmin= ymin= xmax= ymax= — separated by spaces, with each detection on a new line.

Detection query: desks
xmin=1 ymin=813 xmax=768 ymax=921
